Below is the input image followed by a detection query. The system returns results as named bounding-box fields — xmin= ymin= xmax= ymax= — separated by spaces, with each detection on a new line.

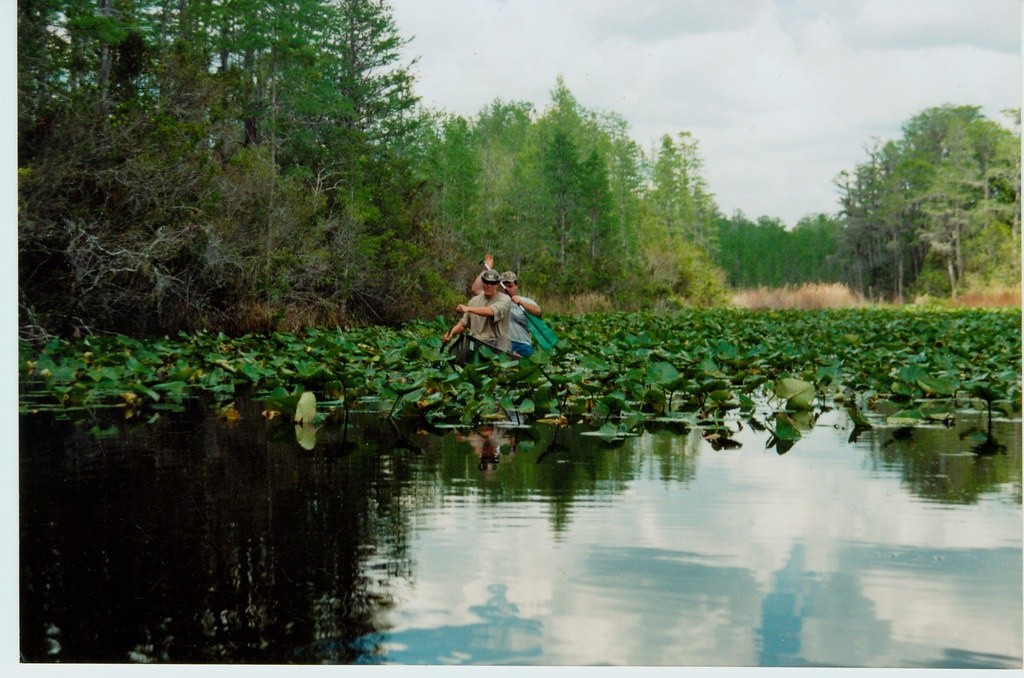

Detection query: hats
xmin=481 ymin=270 xmax=500 ymax=282
xmin=499 ymin=270 xmax=517 ymax=283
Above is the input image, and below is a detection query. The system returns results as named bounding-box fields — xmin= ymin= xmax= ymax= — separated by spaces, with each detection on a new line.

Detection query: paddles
xmin=479 ymin=258 xmax=559 ymax=352
xmin=433 ymin=311 xmax=461 ymax=369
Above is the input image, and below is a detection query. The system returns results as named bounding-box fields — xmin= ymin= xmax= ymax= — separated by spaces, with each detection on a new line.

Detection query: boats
xmin=446 ymin=333 xmax=524 ymax=367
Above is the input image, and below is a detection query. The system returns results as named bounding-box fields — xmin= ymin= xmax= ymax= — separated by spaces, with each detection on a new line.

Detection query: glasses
xmin=481 ymin=277 xmax=500 ymax=285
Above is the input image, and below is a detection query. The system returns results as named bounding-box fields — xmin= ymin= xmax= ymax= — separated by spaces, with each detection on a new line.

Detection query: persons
xmin=442 ymin=270 xmax=512 ymax=355
xmin=471 ymin=254 xmax=542 ymax=361
